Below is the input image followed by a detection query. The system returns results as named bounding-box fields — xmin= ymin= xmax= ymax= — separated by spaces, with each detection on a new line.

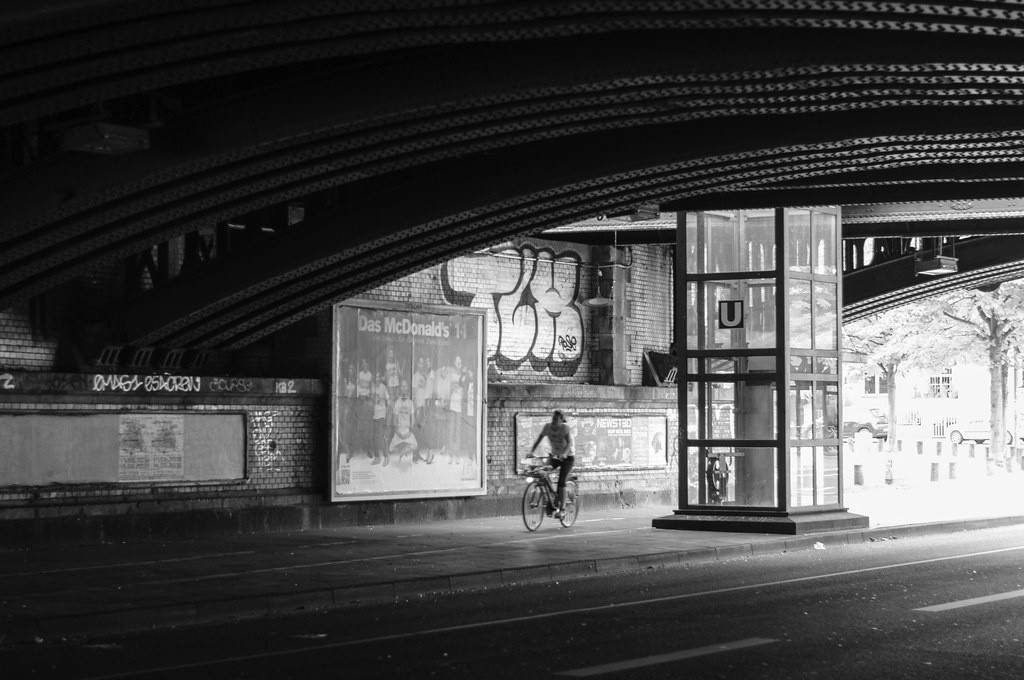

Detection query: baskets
xmin=521 ymin=459 xmax=548 ymax=477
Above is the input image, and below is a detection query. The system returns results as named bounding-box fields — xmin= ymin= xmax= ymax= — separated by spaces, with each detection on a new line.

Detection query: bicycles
xmin=521 ymin=454 xmax=581 ymax=531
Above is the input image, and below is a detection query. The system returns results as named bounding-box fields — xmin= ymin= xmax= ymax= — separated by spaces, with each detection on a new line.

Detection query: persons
xmin=584 ymin=440 xmax=598 ymax=464
xmin=339 ymin=347 xmax=478 ymax=465
xmin=526 ymin=408 xmax=576 ymax=519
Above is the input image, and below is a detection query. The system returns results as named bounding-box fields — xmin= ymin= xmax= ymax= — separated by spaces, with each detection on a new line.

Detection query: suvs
xmin=804 ymin=406 xmax=888 ymax=452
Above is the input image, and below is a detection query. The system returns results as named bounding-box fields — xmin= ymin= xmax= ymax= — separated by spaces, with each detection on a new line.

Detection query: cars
xmin=945 ymin=410 xmax=1024 ymax=445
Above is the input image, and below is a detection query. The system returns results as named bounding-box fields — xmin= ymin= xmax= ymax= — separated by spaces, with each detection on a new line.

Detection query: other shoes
xmin=555 ymin=508 xmax=566 ymax=519
